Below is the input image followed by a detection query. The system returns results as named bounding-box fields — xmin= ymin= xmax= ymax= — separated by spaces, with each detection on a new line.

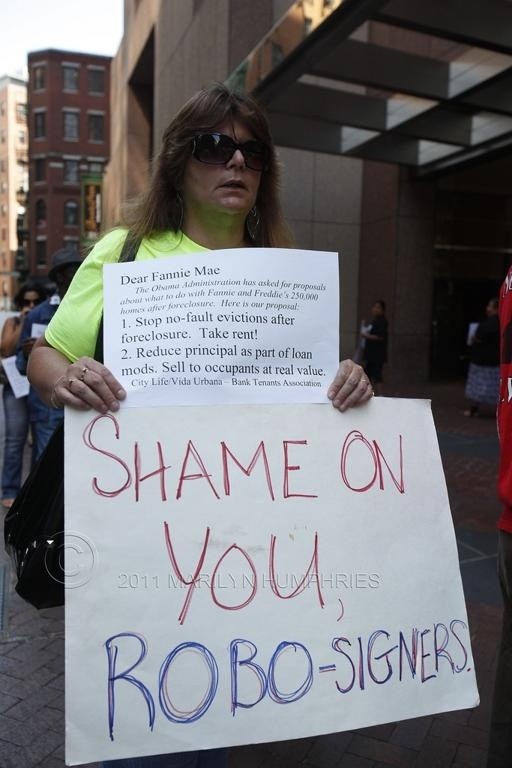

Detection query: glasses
xmin=178 ymin=130 xmax=274 ymax=172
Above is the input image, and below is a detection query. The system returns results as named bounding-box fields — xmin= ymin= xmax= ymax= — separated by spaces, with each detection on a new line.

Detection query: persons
xmin=359 ymin=298 xmax=389 ymax=396
xmin=23 ymin=86 xmax=376 ymax=417
xmin=12 ymin=245 xmax=84 ymax=469
xmin=479 ymin=259 xmax=512 ymax=768
xmin=457 ymin=295 xmax=500 ymax=421
xmin=1 ymin=282 xmax=46 ymax=509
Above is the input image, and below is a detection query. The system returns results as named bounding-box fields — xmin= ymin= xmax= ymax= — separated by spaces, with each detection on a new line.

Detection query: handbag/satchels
xmin=4 ymin=416 xmax=64 ymax=611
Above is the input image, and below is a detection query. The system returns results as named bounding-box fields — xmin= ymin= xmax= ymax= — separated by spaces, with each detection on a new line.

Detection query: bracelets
xmin=50 ymin=376 xmax=64 ymax=411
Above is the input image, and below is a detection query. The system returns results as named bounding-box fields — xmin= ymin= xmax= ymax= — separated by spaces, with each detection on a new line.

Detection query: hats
xmin=47 ymin=246 xmax=83 ymax=282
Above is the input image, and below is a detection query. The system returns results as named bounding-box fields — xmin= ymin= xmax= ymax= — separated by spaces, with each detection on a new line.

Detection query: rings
xmin=80 ymin=368 xmax=89 ymax=381
xmin=69 ymin=378 xmax=78 ymax=391
xmin=360 ymin=379 xmax=370 ymax=386
